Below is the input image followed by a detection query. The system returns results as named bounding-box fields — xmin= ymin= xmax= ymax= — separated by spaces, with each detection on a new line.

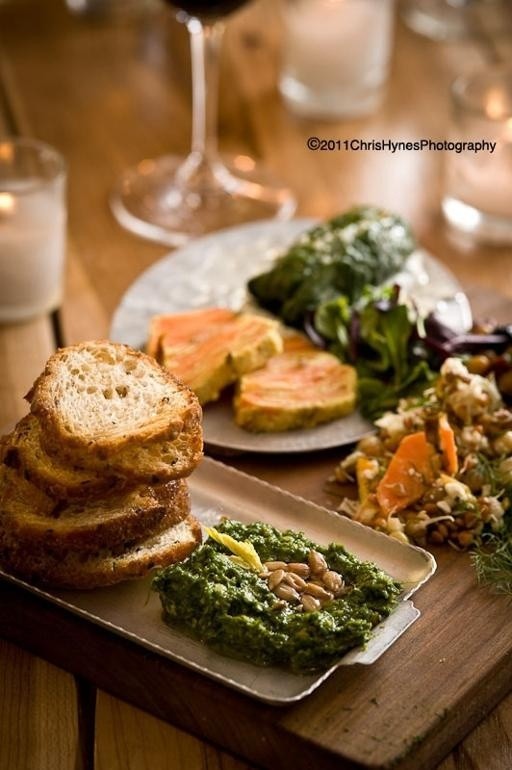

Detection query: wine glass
xmin=107 ymin=0 xmax=291 ymax=246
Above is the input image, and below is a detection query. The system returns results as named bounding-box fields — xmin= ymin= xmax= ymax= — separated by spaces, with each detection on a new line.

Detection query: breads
xmin=0 ymin=340 xmax=204 ymax=590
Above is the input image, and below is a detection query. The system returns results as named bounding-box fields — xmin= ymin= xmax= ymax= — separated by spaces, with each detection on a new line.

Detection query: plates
xmin=110 ymin=219 xmax=473 ymax=456
xmin=0 ymin=459 xmax=439 ymax=706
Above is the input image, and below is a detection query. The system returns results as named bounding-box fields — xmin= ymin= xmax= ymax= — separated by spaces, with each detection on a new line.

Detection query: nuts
xmin=258 ymin=549 xmax=347 ymax=613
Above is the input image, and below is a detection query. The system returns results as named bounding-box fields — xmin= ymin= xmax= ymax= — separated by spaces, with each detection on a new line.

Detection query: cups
xmin=437 ymin=65 xmax=510 ymax=247
xmin=0 ymin=133 xmax=68 ymax=326
xmin=275 ymin=0 xmax=398 ymax=121
